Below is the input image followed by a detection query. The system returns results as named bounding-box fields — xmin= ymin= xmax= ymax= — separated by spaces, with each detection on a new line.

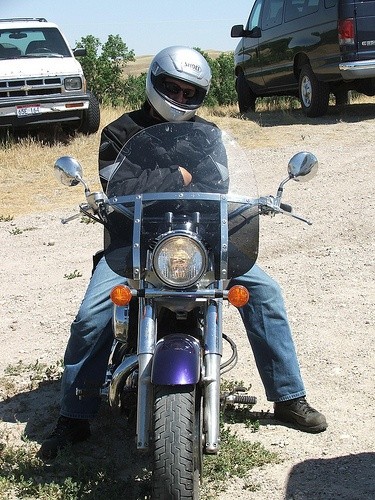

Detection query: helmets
xmin=146 ymin=45 xmax=212 ymax=123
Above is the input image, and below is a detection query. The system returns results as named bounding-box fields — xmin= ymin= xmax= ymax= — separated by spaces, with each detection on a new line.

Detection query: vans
xmin=230 ymin=1 xmax=375 ymax=117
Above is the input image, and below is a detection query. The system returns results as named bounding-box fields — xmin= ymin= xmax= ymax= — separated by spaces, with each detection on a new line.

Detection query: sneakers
xmin=42 ymin=415 xmax=90 ymax=448
xmin=274 ymin=397 xmax=328 ymax=432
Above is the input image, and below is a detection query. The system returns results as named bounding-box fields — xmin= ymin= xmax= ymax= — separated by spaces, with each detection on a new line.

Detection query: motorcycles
xmin=52 ymin=121 xmax=320 ymax=500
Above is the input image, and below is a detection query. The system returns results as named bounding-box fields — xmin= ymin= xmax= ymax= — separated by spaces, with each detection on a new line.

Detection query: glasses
xmin=163 ymin=80 xmax=195 ymax=99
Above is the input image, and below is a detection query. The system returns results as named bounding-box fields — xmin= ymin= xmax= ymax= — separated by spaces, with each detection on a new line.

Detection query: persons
xmin=41 ymin=44 xmax=327 ymax=460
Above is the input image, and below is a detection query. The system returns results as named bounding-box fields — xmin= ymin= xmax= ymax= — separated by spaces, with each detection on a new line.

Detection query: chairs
xmin=278 ymin=6 xmax=298 ymax=17
xmin=25 ymin=40 xmax=51 ymax=54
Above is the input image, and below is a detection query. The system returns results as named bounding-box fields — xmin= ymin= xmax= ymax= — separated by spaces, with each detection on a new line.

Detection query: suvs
xmin=0 ymin=17 xmax=101 ymax=136
